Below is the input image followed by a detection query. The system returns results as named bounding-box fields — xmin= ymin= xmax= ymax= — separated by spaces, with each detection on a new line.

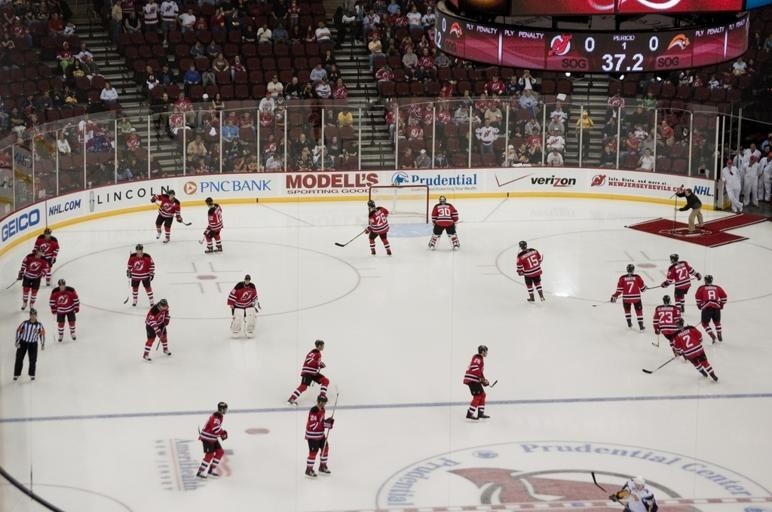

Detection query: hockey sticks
xmin=645 ymin=277 xmax=697 ymax=289
xmin=642 ymin=352 xmax=683 ymax=374
xmin=590 ymin=470 xmax=633 ymax=511
xmin=592 ymin=289 xmax=641 ymax=307
xmin=124 ymin=251 xmax=132 ymax=304
xmin=198 ymin=236 xmax=205 ymax=245
xmin=652 ymin=335 xmax=659 ymax=348
xmin=153 ymin=201 xmax=192 ymax=226
xmin=489 ymin=380 xmax=498 ymax=388
xmin=334 ymin=227 xmax=365 ymax=247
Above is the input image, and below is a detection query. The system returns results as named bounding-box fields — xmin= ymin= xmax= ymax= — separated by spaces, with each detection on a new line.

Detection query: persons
xmin=660 ymin=254 xmax=703 ymax=312
xmin=427 ymin=195 xmax=463 ymax=252
xmin=33 ymin=228 xmax=59 ymax=287
xmin=600 ymin=21 xmax=761 ymax=173
xmin=1 ymin=1 xmax=160 ymax=219
xmin=288 ymin=339 xmax=330 ymax=407
xmin=360 ymin=0 xmax=572 ymax=170
xmin=722 ymin=160 xmax=743 ymax=213
xmin=304 ymin=395 xmax=332 ymax=476
xmin=741 ymin=156 xmax=761 ymax=208
xmin=695 ymin=274 xmax=727 ymax=345
xmin=203 ymin=197 xmax=225 ymax=253
xmin=143 ymin=298 xmax=172 ymax=362
xmin=227 ymin=275 xmax=262 ymax=338
xmin=575 ymin=112 xmax=592 ymax=160
xmin=609 ymin=264 xmax=647 ymax=331
xmin=126 ymin=243 xmax=155 ymax=308
xmin=365 ymin=200 xmax=392 ymax=258
xmin=13 ymin=308 xmax=45 ymax=382
xmin=49 ymin=279 xmax=80 ymax=342
xmin=653 ymin=295 xmax=686 ymax=356
xmin=517 ymin=240 xmax=544 ymax=302
xmin=17 ymin=248 xmax=51 ymax=309
xmin=160 ymin=0 xmax=359 ymax=177
xmin=672 ymin=318 xmax=720 ymax=382
xmin=196 ymin=402 xmax=228 ymax=480
xmin=758 ymin=133 xmax=772 ymax=202
xmin=150 ymin=189 xmax=182 ymax=243
xmin=674 ymin=188 xmax=704 ymax=233
xmin=462 ymin=345 xmax=489 ymax=419
xmin=608 ymin=476 xmax=658 ymax=512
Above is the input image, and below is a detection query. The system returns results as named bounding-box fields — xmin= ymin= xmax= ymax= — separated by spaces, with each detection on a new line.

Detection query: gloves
xmin=695 ymin=272 xmax=701 ymax=281
xmin=517 ymin=270 xmax=524 ymax=275
xmin=176 ymin=215 xmax=182 ymax=222
xmin=17 ymin=271 xmax=23 ymax=280
xmin=611 ymin=295 xmax=617 ymax=302
xmin=164 ymin=316 xmax=170 ymax=325
xmin=52 ymin=309 xmax=57 ymax=314
xmin=661 ymin=283 xmax=667 ymax=288
xmin=720 ymin=302 xmax=724 ymax=309
xmin=157 ymin=331 xmax=163 ymax=338
xmin=655 ymin=330 xmax=660 ymax=335
xmin=697 ymin=303 xmax=701 ymax=309
xmin=483 ymin=380 xmax=489 ymax=386
xmin=220 ymin=431 xmax=227 ymax=440
xmin=323 ymin=421 xmax=332 ymax=429
xmin=73 ymin=306 xmax=79 ymax=313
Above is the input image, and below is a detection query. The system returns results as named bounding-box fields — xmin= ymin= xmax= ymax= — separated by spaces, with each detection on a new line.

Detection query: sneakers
xmin=156 ymin=233 xmax=161 ymax=239
xmin=21 ymin=304 xmax=27 ymax=310
xmin=708 ymin=331 xmax=715 ymax=344
xmin=699 ymin=368 xmax=708 ymax=377
xmin=163 ymin=350 xmax=171 ymax=356
xmin=58 ymin=335 xmax=63 ymax=341
xmin=305 ymin=467 xmax=317 ymax=477
xmin=30 ymin=303 xmax=33 ymax=308
xmin=681 ymin=303 xmax=684 ymax=312
xmin=163 ymin=239 xmax=169 ymax=243
xmin=709 ymin=371 xmax=717 ymax=381
xmin=319 ymin=466 xmax=330 ymax=473
xmin=205 ymin=248 xmax=213 ymax=253
xmin=197 ymin=471 xmax=207 ymax=478
xmin=387 ymin=249 xmax=391 ymax=255
xmin=627 ymin=319 xmax=632 ymax=327
xmin=466 ymin=413 xmax=479 ymax=420
xmin=30 ymin=376 xmax=35 ymax=380
xmin=214 ymin=246 xmax=222 ymax=252
xmin=209 ymin=469 xmax=219 ymax=476
xmin=143 ymin=355 xmax=151 ymax=361
xmin=527 ymin=294 xmax=534 ymax=301
xmin=13 ymin=375 xmax=19 ymax=380
xmin=538 ymin=290 xmax=543 ymax=298
xmin=71 ymin=334 xmax=76 ymax=340
xmin=638 ymin=321 xmax=645 ymax=330
xmin=717 ymin=331 xmax=722 ymax=342
xmin=478 ymin=412 xmax=489 ymax=418
xmin=371 ymin=248 xmax=375 ymax=255
xmin=149 ymin=301 xmax=154 ymax=307
xmin=132 ymin=301 xmax=137 ymax=306
